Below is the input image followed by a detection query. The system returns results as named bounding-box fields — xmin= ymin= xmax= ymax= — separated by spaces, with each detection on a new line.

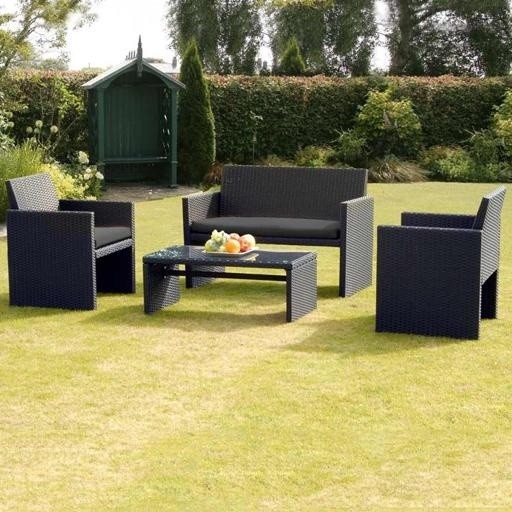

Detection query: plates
xmin=202 ymin=248 xmax=258 ymax=257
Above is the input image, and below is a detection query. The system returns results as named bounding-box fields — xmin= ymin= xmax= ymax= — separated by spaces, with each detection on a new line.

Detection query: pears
xmin=223 ymin=240 xmax=241 ymax=253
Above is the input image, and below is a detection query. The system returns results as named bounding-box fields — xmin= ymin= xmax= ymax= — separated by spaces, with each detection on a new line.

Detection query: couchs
xmin=4 ymin=172 xmax=135 ymax=311
xmin=181 ymin=162 xmax=374 ymax=299
xmin=374 ymin=184 xmax=507 ymax=342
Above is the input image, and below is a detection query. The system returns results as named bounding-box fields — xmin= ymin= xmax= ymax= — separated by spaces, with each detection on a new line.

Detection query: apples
xmin=243 ymin=234 xmax=256 ymax=248
xmin=238 ymin=236 xmax=252 ymax=252
xmin=230 ymin=233 xmax=240 ymax=241
